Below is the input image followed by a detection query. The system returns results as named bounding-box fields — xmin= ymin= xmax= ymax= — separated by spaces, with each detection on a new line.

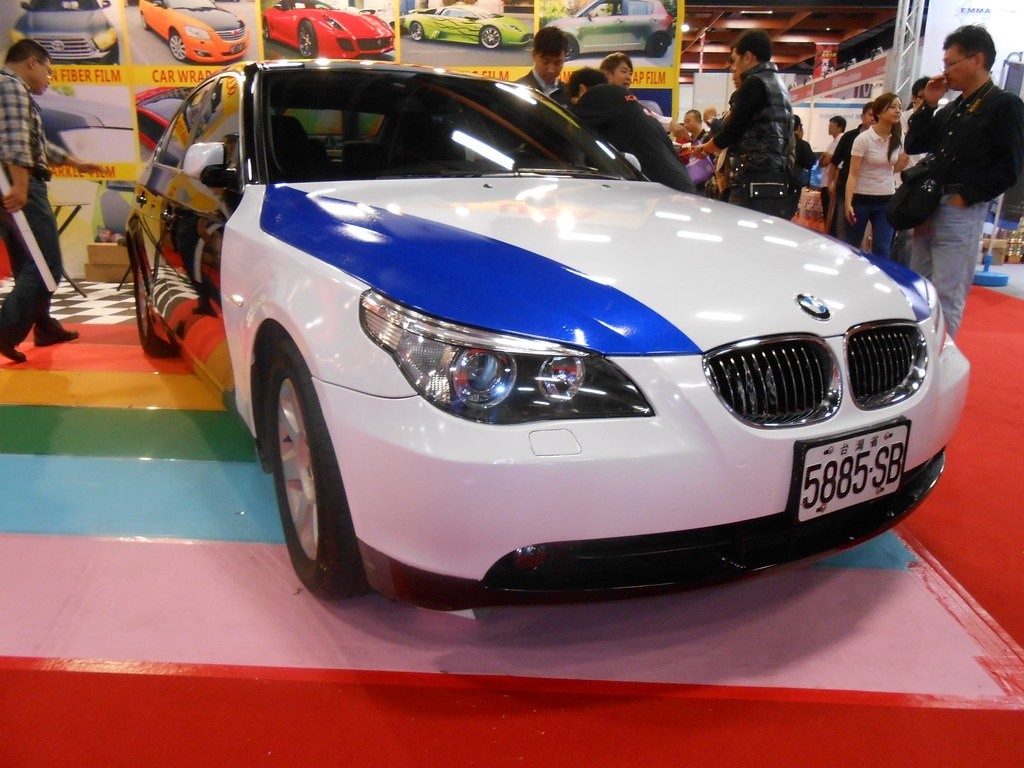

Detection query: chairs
xmin=371 ymin=79 xmax=457 ymax=173
xmin=271 ymin=115 xmax=334 ymax=180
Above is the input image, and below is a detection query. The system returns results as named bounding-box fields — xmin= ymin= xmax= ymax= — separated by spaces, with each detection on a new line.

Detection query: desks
xmin=52 ymin=201 xmax=90 ymax=299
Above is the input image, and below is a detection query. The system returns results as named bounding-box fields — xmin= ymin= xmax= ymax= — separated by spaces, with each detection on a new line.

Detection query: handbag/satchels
xmin=886 ymin=158 xmax=941 ymax=231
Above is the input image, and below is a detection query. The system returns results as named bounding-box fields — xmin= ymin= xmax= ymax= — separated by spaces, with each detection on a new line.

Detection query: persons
xmin=827 ymin=73 xmax=934 ymax=257
xmin=886 ymin=21 xmax=1024 ymax=343
xmin=124 ymin=133 xmax=241 ymax=322
xmin=510 ymin=27 xmax=696 ymax=194
xmin=669 ymin=28 xmax=794 ymax=222
xmin=795 ymin=114 xmax=847 ymax=230
xmin=0 ymin=37 xmax=80 ymax=363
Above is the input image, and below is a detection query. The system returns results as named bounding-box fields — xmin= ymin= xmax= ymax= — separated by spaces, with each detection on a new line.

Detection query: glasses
xmin=32 ymin=56 xmax=53 ymax=79
xmin=945 ymin=55 xmax=974 ymax=68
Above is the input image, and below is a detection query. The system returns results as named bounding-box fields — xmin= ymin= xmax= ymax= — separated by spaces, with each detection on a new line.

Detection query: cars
xmin=545 ymin=0 xmax=673 ymax=60
xmin=126 ymin=59 xmax=972 ymax=612
xmin=405 ymin=6 xmax=531 ymax=49
xmin=263 ymin=0 xmax=395 ymax=59
xmin=139 ymin=0 xmax=250 ymax=63
xmin=9 ymin=0 xmax=119 ymax=65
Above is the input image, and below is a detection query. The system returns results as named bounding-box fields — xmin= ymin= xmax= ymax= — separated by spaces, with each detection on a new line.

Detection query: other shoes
xmin=34 ymin=327 xmax=79 ymax=346
xmin=0 ymin=345 xmax=26 ymax=363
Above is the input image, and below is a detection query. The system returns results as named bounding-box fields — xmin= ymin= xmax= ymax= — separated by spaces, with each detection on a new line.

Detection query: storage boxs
xmin=85 ymin=243 xmax=135 ymax=283
